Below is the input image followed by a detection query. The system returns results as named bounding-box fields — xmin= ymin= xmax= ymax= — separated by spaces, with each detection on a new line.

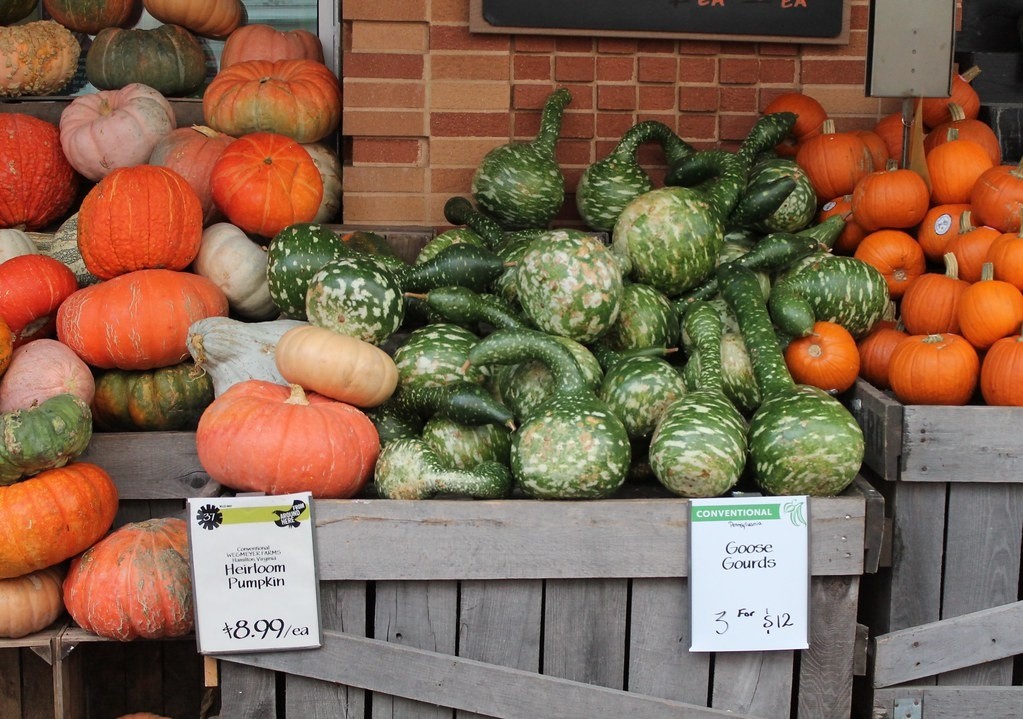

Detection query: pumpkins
xmin=0 ymin=0 xmax=1022 ymax=642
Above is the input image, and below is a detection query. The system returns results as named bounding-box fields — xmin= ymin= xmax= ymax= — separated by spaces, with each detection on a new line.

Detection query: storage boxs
xmin=845 ymin=376 xmax=1023 ymax=719
xmin=213 ymin=466 xmax=889 ymax=717
xmin=1 ymin=424 xmax=227 ymax=719
